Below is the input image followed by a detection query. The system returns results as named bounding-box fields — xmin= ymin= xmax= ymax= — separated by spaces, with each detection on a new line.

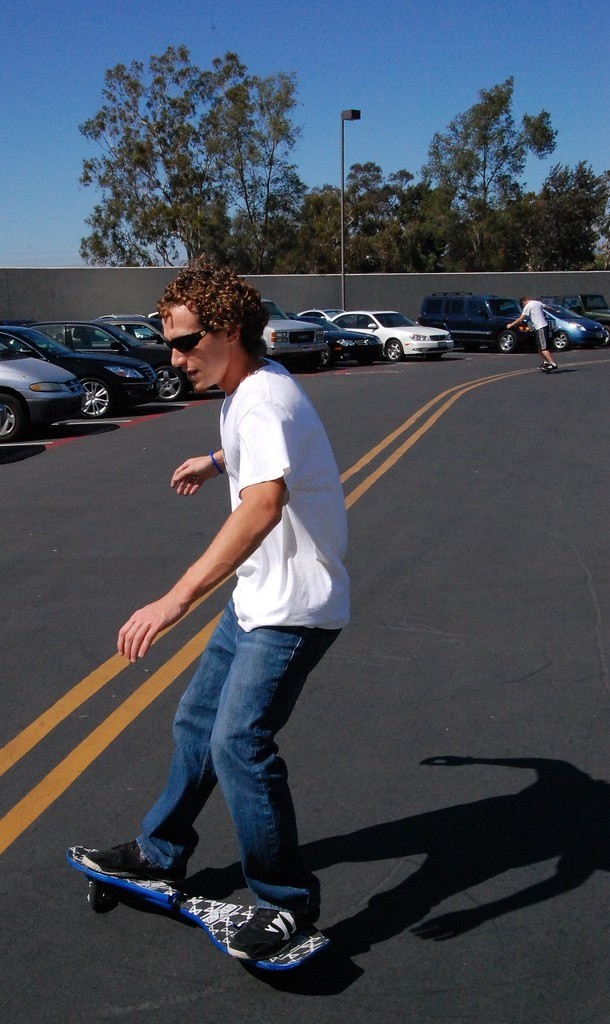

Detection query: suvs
xmin=98 ymin=313 xmax=164 ymax=333
xmin=539 ymin=294 xmax=610 ymax=347
xmin=258 ymin=297 xmax=327 ymax=374
xmin=417 ymin=292 xmax=556 ymax=354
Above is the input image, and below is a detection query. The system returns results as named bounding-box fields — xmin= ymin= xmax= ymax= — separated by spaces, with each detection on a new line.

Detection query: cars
xmin=291 ymin=317 xmax=382 ymax=368
xmin=330 ymin=311 xmax=454 ymax=362
xmin=26 ymin=319 xmax=194 ymax=402
xmin=109 ymin=321 xmax=167 ymax=346
xmin=0 ymin=326 xmax=161 ymax=419
xmin=297 ymin=308 xmax=347 ymax=321
xmin=541 ymin=302 xmax=607 ymax=352
xmin=0 ymin=341 xmax=87 ymax=443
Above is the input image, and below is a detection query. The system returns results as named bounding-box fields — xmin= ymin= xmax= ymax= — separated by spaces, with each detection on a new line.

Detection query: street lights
xmin=341 ymin=109 xmax=362 ymax=311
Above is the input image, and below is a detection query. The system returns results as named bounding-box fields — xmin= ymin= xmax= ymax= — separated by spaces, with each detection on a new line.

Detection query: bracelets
xmin=208 ymin=450 xmax=223 ymax=474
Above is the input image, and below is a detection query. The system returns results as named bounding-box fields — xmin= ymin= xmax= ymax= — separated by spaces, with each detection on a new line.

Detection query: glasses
xmin=166 ymin=327 xmax=213 ymax=354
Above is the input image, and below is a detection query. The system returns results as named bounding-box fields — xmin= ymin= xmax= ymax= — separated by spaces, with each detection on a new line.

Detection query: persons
xmin=81 ymin=257 xmax=351 ymax=962
xmin=506 ymin=296 xmax=561 ymax=369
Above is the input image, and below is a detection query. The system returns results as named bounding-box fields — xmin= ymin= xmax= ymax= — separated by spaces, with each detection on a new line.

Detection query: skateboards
xmin=536 ymin=366 xmax=558 ymax=374
xmin=67 ymin=845 xmax=330 ymax=972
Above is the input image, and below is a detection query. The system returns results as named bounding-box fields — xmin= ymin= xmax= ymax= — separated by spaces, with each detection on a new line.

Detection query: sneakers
xmin=537 ymin=362 xmax=550 ymax=370
xmin=82 ymin=839 xmax=186 ymax=881
xmin=545 ymin=364 xmax=559 ymax=371
xmin=227 ymin=907 xmax=321 ymax=960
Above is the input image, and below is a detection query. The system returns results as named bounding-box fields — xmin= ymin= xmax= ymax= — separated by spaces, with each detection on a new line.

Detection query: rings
xmin=192 ymin=475 xmax=196 ymax=479
